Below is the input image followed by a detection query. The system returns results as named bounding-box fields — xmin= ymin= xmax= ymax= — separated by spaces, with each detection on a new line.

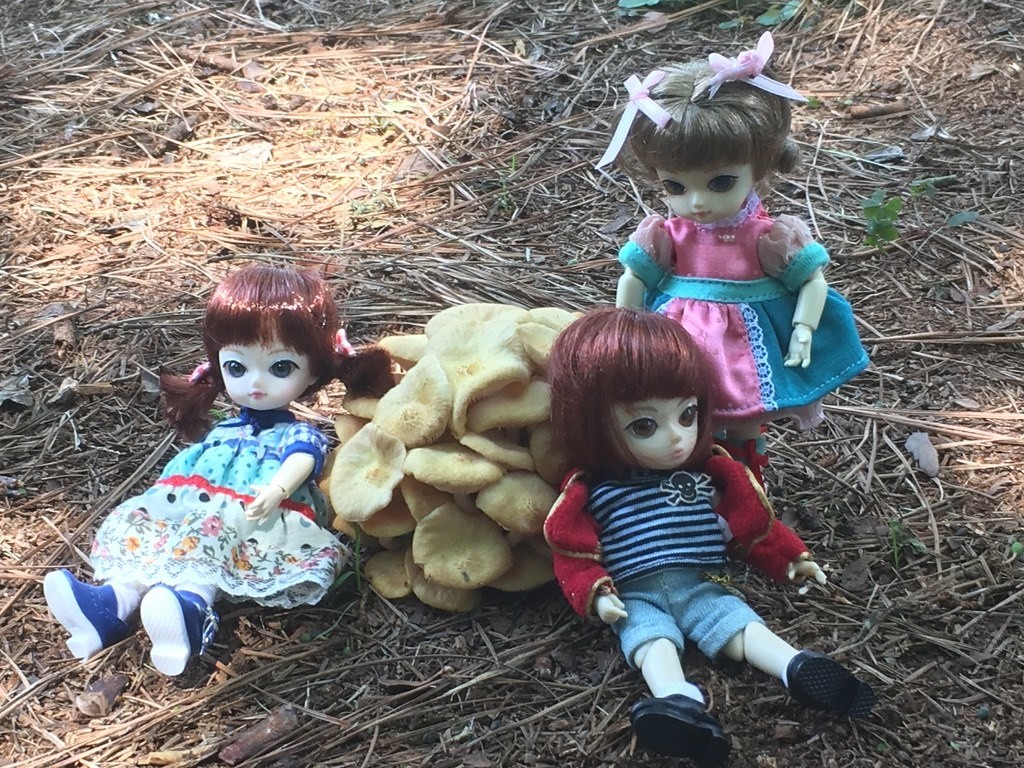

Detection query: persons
xmin=42 ymin=266 xmax=398 ymax=678
xmin=543 ymin=307 xmax=875 ymax=766
xmin=592 ymin=29 xmax=871 ymax=495
